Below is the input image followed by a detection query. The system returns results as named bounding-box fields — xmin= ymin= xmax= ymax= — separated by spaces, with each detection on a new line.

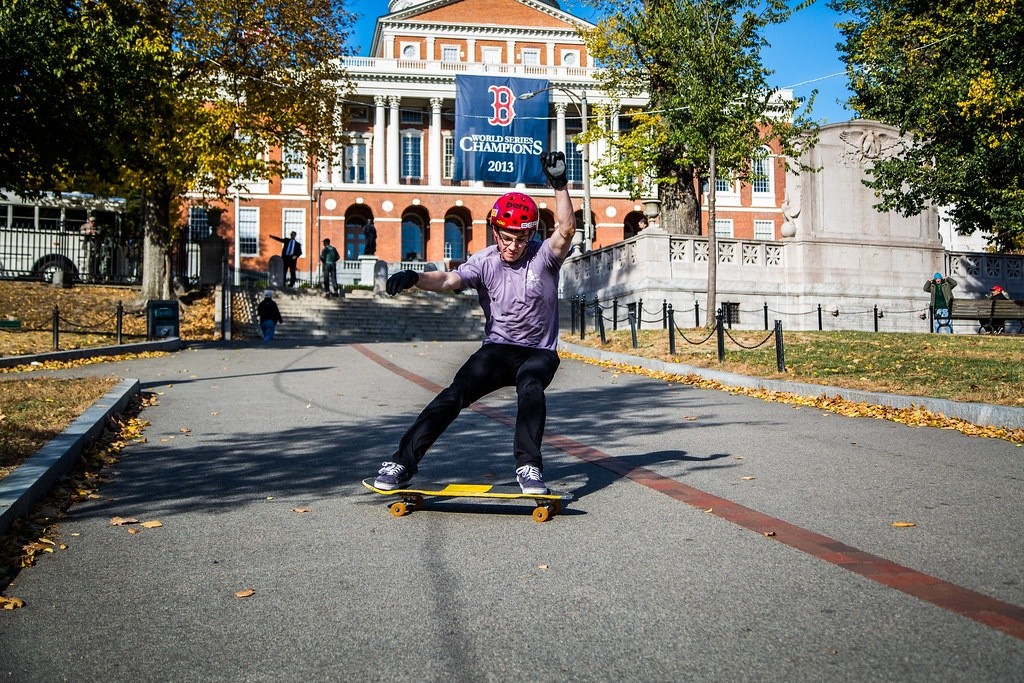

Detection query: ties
xmin=288 ymin=240 xmax=293 ymax=254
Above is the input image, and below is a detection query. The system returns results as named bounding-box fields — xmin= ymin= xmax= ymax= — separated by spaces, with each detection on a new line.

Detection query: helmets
xmin=491 ymin=191 xmax=539 ymax=232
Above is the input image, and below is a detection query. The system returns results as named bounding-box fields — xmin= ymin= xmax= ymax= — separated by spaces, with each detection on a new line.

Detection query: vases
xmin=643 ymin=200 xmax=662 ymax=222
xmin=571 ymin=229 xmax=585 ymax=248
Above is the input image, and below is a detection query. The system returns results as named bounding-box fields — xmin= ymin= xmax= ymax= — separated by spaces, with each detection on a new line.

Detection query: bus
xmin=0 ymin=187 xmax=147 ymax=289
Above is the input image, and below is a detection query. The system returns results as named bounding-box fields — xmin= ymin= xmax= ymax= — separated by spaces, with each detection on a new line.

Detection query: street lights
xmin=517 ymin=83 xmax=595 ymax=253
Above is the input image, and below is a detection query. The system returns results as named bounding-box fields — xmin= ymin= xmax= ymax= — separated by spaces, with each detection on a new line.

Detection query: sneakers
xmin=516 ymin=464 xmax=547 ymax=494
xmin=374 ymin=461 xmax=413 ymax=490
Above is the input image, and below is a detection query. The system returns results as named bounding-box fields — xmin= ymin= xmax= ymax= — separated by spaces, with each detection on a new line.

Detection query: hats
xmin=265 ymin=291 xmax=273 ymax=298
xmin=322 ymin=238 xmax=330 ymax=244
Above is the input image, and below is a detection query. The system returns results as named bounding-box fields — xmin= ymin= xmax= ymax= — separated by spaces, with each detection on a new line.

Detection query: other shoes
xmin=288 ymin=283 xmax=293 ymax=287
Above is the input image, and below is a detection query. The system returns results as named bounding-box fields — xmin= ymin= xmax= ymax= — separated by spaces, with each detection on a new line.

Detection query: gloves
xmin=386 ymin=269 xmax=419 ymax=296
xmin=540 ymin=151 xmax=568 ymax=189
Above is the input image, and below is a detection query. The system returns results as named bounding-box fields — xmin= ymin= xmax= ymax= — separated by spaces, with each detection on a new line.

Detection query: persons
xmin=320 ymin=238 xmax=340 ymax=297
xmin=638 ymin=218 xmax=648 ymax=229
xmin=269 ymin=231 xmax=302 ymax=293
xmin=258 ymin=292 xmax=283 ymax=345
xmin=373 ymin=151 xmax=575 ymax=494
xmin=979 ymin=286 xmax=1007 ymax=335
xmin=924 ymin=272 xmax=957 ymax=334
xmin=80 ymin=217 xmax=101 ymax=235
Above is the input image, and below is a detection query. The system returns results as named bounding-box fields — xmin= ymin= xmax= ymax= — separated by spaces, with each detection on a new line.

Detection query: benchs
xmin=934 ymin=297 xmax=1024 ymax=335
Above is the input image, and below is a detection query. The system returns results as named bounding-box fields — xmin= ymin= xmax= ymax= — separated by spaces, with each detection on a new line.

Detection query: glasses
xmin=495 ymin=225 xmax=531 ymax=244
